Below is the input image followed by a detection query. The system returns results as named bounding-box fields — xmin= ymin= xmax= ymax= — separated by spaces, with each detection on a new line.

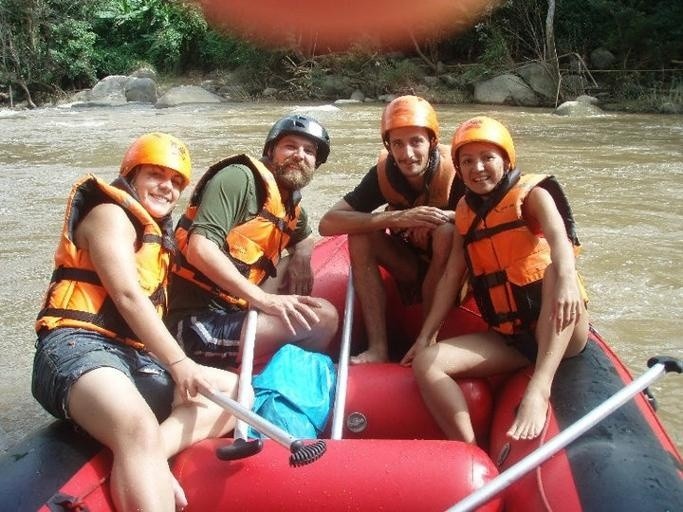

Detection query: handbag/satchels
xmin=167 ymin=357 xmax=189 ymax=367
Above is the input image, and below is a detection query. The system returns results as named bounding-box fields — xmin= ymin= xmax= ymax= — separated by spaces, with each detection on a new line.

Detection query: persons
xmin=401 ymin=115 xmax=590 ymax=448
xmin=31 ymin=131 xmax=255 ymax=511
xmin=163 ymin=114 xmax=338 ymax=369
xmin=319 ymin=96 xmax=456 ymax=364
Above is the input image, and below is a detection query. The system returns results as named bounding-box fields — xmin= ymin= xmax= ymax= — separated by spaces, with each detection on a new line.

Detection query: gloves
xmin=0 ymin=232 xmax=682 ymax=511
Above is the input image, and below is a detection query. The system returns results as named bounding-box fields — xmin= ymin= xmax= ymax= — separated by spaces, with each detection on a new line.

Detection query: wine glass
xmin=570 ymin=311 xmax=576 ymax=315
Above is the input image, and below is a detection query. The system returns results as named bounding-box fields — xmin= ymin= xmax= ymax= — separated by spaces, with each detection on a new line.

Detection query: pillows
xmin=118 ymin=131 xmax=195 ymax=190
xmin=379 ymin=93 xmax=442 ymax=148
xmin=449 ymin=114 xmax=517 ymax=175
xmin=263 ymin=109 xmax=333 ymax=172
